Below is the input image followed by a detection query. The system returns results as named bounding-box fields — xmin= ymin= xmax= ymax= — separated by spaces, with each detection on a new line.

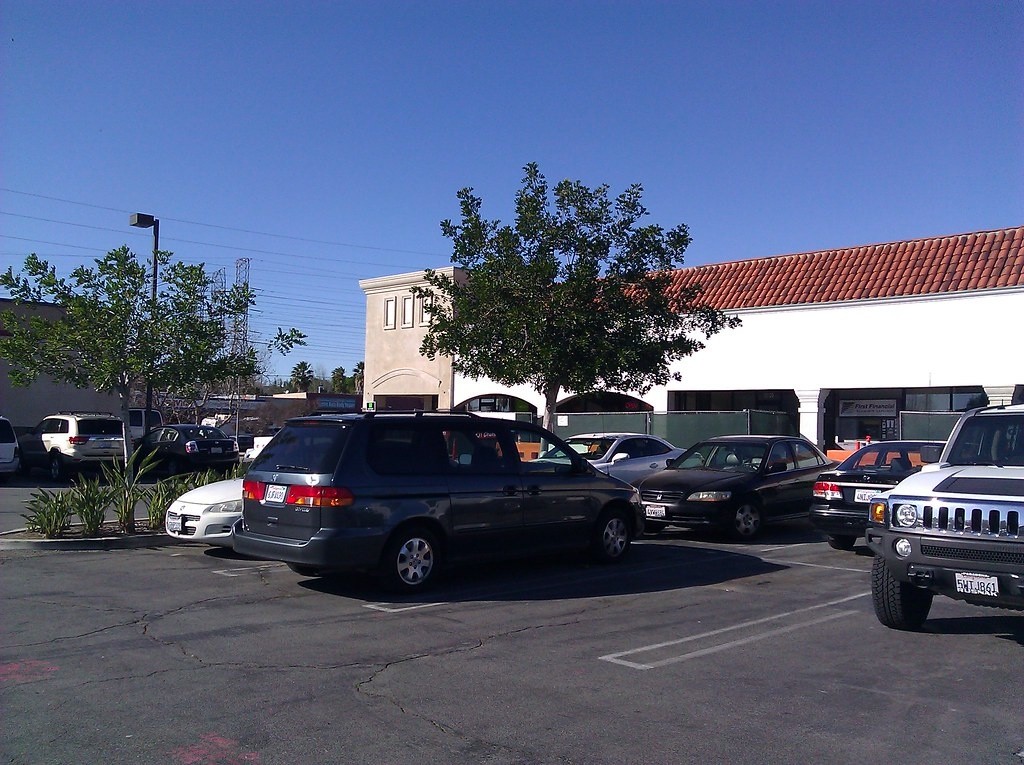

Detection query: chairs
xmin=407 ymin=429 xmax=451 ymax=475
xmin=721 ymin=451 xmax=743 ymax=470
xmin=618 ymin=439 xmax=642 ymax=460
xmin=471 ymin=448 xmax=497 ymax=473
xmin=1010 ymin=428 xmax=1024 ymax=456
xmin=645 ymin=444 xmax=663 ymax=457
xmin=890 ymin=457 xmax=914 ymax=472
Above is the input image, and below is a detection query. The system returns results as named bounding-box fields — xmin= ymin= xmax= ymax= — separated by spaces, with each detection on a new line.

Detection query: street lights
xmin=130 ymin=213 xmax=159 ymax=459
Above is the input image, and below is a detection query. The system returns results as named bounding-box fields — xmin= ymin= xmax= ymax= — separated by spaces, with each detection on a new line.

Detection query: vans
xmin=232 ymin=409 xmax=647 ymax=593
xmin=129 ymin=409 xmax=163 ymax=446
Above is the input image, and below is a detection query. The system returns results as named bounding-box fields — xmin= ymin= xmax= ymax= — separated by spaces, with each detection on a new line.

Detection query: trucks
xmin=201 ymin=415 xmax=244 ymax=436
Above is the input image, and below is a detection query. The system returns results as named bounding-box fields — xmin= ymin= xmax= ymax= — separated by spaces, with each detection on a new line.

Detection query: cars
xmin=527 ymin=432 xmax=705 ymax=492
xmin=134 ymin=424 xmax=240 ymax=478
xmin=257 ymin=427 xmax=281 ymax=436
xmin=229 ymin=436 xmax=253 ymax=452
xmin=166 ymin=479 xmax=243 ymax=548
xmin=809 ymin=440 xmax=979 ymax=551
xmin=636 ymin=435 xmax=843 ymax=541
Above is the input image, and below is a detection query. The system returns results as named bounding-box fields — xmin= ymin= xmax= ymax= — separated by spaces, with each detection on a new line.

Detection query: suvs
xmin=865 ymin=401 xmax=1024 ymax=630
xmin=16 ymin=411 xmax=135 ymax=483
xmin=0 ymin=416 xmax=19 ymax=481
